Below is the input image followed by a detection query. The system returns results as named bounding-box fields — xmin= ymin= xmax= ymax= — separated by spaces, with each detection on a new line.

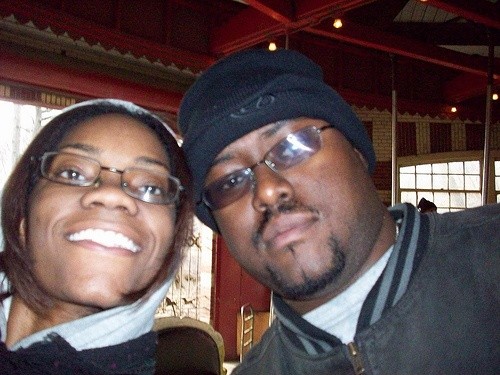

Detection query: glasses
xmin=198 ymin=123 xmax=341 ymax=210
xmin=36 ymin=149 xmax=184 ymax=207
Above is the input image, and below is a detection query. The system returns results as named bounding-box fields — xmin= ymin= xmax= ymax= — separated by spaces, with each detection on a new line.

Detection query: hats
xmin=180 ymin=47 xmax=378 ymax=230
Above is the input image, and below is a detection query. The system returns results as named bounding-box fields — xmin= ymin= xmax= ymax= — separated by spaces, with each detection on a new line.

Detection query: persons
xmin=417 ymin=199 xmax=437 ymax=214
xmin=0 ymin=100 xmax=193 ymax=375
xmin=177 ymin=48 xmax=500 ymax=375
